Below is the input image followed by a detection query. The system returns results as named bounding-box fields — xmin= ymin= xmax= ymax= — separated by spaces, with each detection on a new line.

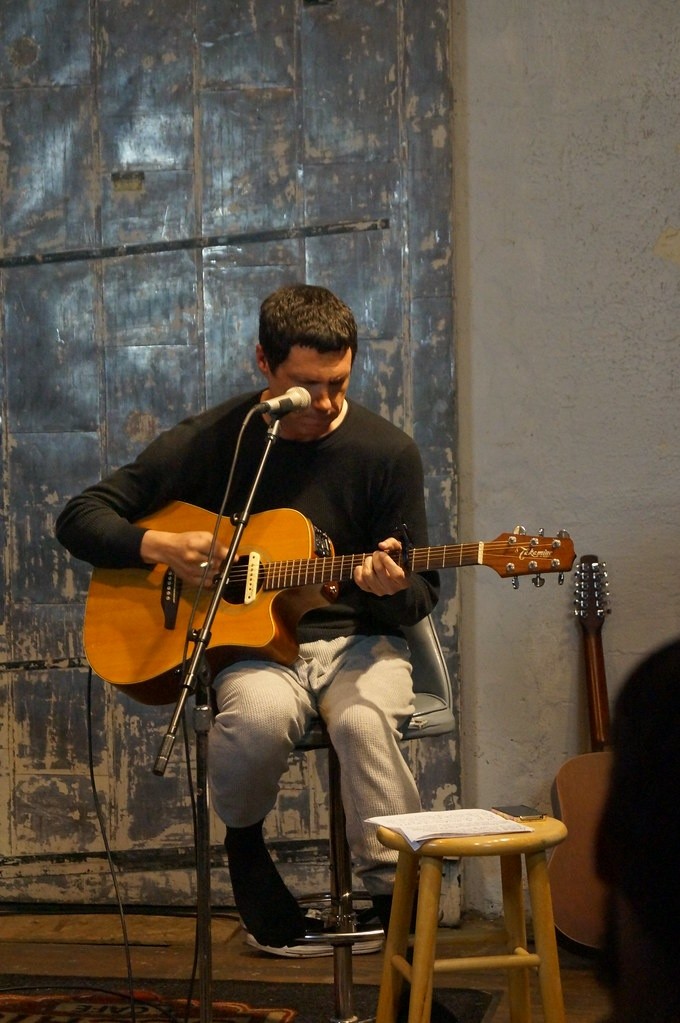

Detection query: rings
xmin=203 ymin=560 xmax=214 ymax=570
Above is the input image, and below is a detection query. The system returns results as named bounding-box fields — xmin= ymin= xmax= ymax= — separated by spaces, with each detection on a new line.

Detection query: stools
xmin=375 ymin=810 xmax=571 ymax=1023
xmin=275 ymin=610 xmax=448 ymax=1023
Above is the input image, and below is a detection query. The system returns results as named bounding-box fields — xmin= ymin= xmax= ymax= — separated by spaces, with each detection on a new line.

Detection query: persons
xmin=54 ymin=282 xmax=439 ymax=958
xmin=596 ymin=636 xmax=679 ymax=1023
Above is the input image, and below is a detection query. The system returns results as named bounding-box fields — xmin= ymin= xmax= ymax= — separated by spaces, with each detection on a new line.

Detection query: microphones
xmin=251 ymin=386 xmax=311 ymax=414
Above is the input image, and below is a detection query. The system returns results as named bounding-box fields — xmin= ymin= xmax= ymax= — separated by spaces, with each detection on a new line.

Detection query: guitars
xmin=83 ymin=498 xmax=579 ymax=705
xmin=541 ymin=552 xmax=617 ymax=951
